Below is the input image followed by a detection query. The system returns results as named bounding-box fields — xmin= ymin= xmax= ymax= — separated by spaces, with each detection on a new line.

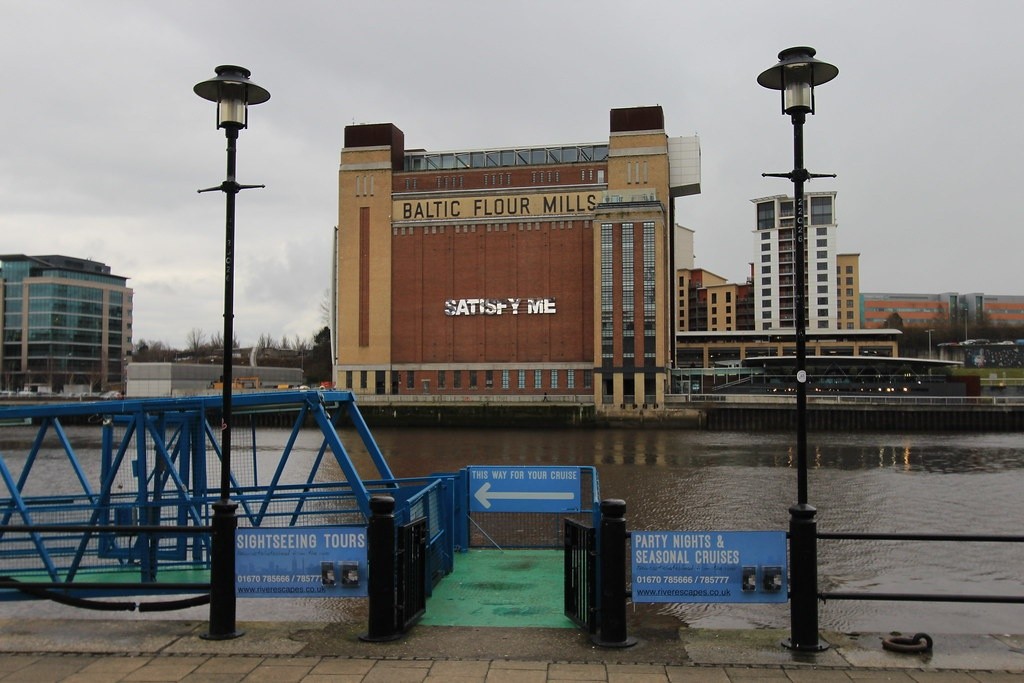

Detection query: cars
xmin=0 ymin=389 xmax=122 ymax=399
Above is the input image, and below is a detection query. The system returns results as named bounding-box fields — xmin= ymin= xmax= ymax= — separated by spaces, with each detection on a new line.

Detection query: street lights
xmin=964 ymin=308 xmax=968 ymax=345
xmin=193 ymin=64 xmax=274 ymax=643
xmin=925 ymin=329 xmax=934 ymax=359
xmin=757 ymin=46 xmax=841 ymax=657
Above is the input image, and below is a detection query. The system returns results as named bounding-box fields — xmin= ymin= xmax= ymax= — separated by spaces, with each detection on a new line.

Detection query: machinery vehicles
xmin=208 ymin=375 xmax=294 ymax=392
